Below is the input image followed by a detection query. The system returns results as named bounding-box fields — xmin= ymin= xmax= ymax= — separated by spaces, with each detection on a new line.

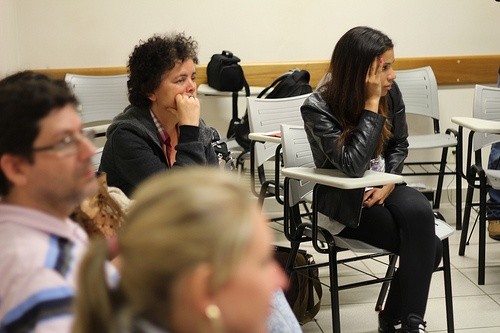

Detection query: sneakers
xmin=378 ymin=311 xmax=427 ymax=333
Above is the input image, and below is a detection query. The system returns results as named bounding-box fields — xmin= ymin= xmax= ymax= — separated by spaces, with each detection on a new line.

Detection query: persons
xmin=0 ymin=69 xmax=97 ymax=333
xmin=97 ymin=32 xmax=221 ymax=199
xmin=70 ymin=165 xmax=290 ymax=333
xmin=487 ymin=66 xmax=500 ymax=240
xmin=300 ymin=25 xmax=444 ymax=333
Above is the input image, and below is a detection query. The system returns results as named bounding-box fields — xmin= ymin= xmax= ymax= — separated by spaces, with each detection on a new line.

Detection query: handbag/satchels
xmin=272 ymin=245 xmax=322 ymax=325
xmin=206 ymin=50 xmax=244 ymax=91
xmin=69 ymin=172 xmax=131 ymax=241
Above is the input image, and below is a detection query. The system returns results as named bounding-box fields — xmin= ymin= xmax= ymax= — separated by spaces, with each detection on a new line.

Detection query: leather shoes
xmin=488 ymin=220 xmax=500 ymax=239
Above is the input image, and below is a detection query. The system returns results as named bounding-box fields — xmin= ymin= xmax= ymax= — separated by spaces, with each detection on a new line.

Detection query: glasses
xmin=31 ymin=129 xmax=95 ymax=158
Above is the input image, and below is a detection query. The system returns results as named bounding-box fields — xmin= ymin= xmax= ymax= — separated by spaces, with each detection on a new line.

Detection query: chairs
xmin=63 ymin=66 xmax=499 ymax=333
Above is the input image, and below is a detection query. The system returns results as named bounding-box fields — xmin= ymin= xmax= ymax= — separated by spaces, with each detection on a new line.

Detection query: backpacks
xmin=236 ymin=68 xmax=313 ymax=148
